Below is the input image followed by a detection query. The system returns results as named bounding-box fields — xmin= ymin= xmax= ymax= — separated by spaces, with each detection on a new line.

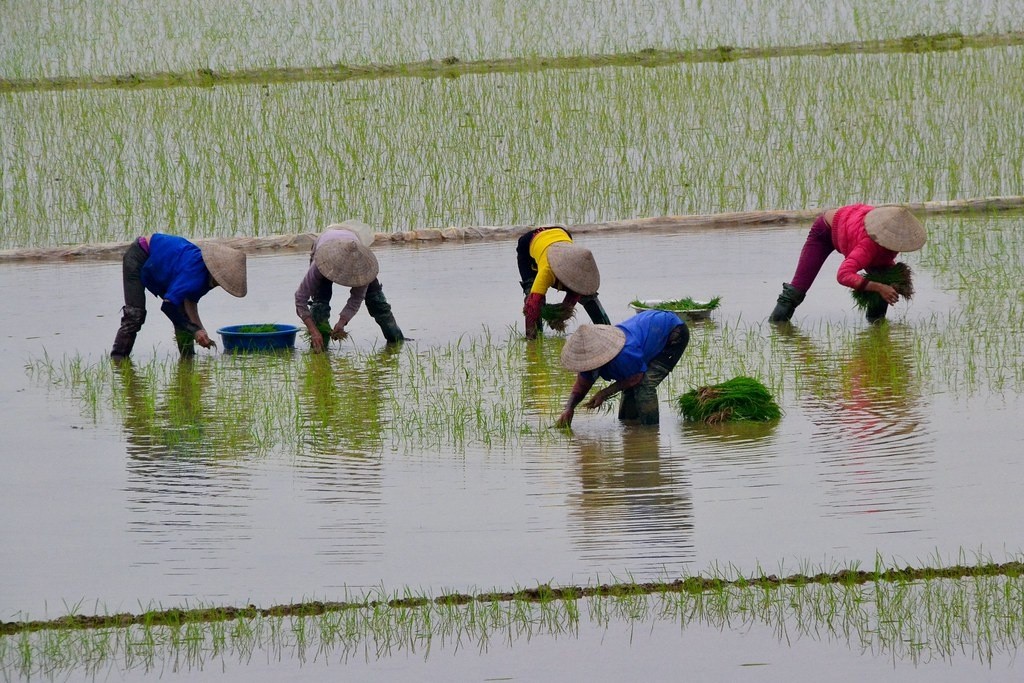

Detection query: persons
xmin=556 ymin=309 xmax=690 ymax=431
xmin=110 ymin=234 xmax=247 ymax=369
xmin=768 ymin=204 xmax=927 ymax=324
xmin=516 ymin=227 xmax=611 ymax=342
xmin=295 ymin=224 xmax=404 ymax=354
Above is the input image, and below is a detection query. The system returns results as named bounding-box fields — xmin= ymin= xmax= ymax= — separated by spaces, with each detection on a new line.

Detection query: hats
xmin=313 ymin=240 xmax=378 ymax=286
xmin=864 ymin=205 xmax=927 ymax=252
xmin=200 ymin=240 xmax=248 ymax=298
xmin=559 ymin=323 xmax=626 ymax=372
xmin=546 ymin=242 xmax=601 ymax=295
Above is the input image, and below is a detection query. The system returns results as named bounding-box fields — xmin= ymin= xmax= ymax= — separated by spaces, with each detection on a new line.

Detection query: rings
xmin=890 ymin=301 xmax=893 ymax=304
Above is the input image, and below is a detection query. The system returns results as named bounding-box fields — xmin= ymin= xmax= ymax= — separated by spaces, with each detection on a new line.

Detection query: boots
xmin=619 ymin=388 xmax=635 ymax=418
xmin=365 ymin=289 xmax=403 ymax=343
xmin=110 ymin=305 xmax=146 ymax=355
xmin=768 ymin=283 xmax=807 ymax=321
xmin=521 ymin=278 xmax=542 ymax=331
xmin=865 ymin=302 xmax=888 ymax=322
xmin=635 ymin=361 xmax=673 ymax=426
xmin=307 ymin=301 xmax=330 ymax=347
xmin=173 ymin=324 xmax=194 ymax=356
xmin=584 ymin=297 xmax=611 ymax=326
